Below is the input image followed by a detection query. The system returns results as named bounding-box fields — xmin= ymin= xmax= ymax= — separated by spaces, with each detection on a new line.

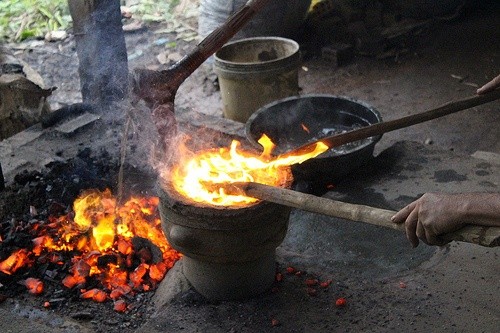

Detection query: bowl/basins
xmin=244 ymin=94 xmax=385 ymax=184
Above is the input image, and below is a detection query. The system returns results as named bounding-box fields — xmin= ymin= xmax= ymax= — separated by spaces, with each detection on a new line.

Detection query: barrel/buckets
xmin=212 ymin=36 xmax=304 ymax=123
